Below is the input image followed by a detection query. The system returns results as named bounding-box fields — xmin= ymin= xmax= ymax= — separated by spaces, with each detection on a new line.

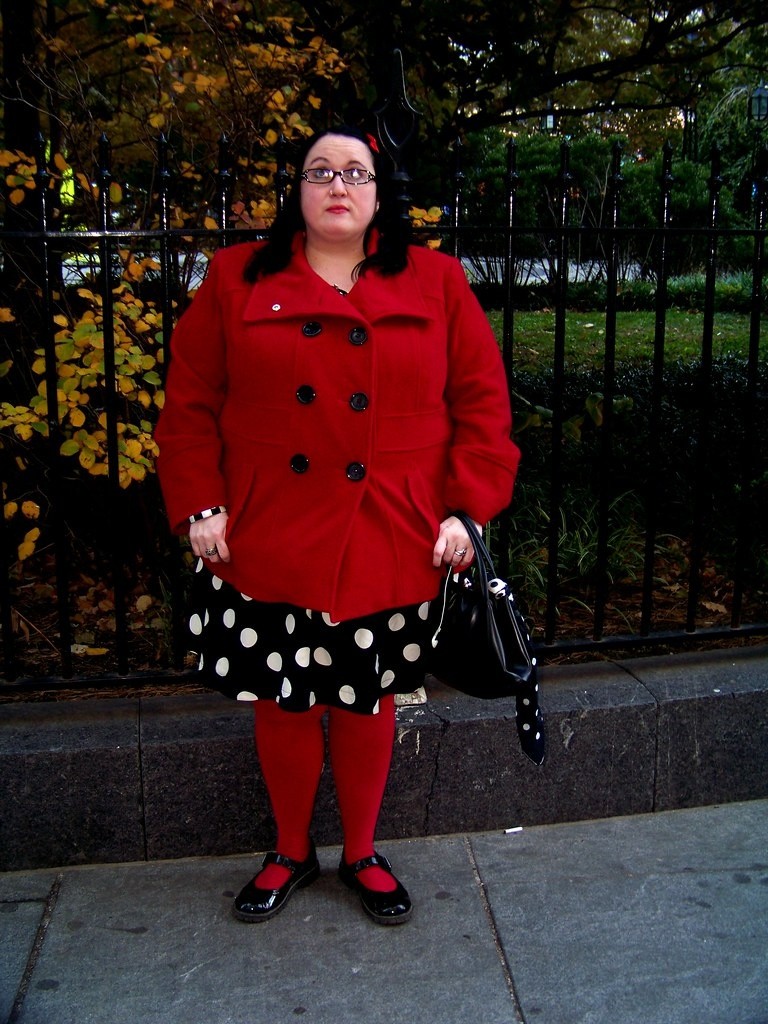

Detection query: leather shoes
xmin=231 ymin=835 xmax=320 ymax=922
xmin=336 ymin=846 xmax=414 ymax=925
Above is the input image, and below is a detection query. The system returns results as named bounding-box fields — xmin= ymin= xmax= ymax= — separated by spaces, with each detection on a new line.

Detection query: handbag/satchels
xmin=424 ymin=509 xmax=535 ymax=700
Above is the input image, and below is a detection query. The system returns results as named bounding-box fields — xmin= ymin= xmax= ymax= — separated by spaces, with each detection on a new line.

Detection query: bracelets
xmin=185 ymin=502 xmax=226 ymax=524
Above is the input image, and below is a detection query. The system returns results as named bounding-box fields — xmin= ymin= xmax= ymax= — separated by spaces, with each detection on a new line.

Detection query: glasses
xmin=296 ymin=168 xmax=376 ymax=185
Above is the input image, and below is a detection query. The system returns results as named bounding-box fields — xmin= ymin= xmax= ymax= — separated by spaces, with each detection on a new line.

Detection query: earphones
xmin=432 ymin=628 xmax=441 ymax=648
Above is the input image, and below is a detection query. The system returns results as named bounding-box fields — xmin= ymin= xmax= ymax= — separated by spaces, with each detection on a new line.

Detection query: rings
xmin=454 ymin=549 xmax=466 ymax=557
xmin=205 ymin=547 xmax=216 ymax=555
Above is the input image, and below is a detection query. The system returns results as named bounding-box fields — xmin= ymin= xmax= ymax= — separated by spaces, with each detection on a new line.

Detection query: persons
xmin=152 ymin=119 xmax=523 ymax=922
